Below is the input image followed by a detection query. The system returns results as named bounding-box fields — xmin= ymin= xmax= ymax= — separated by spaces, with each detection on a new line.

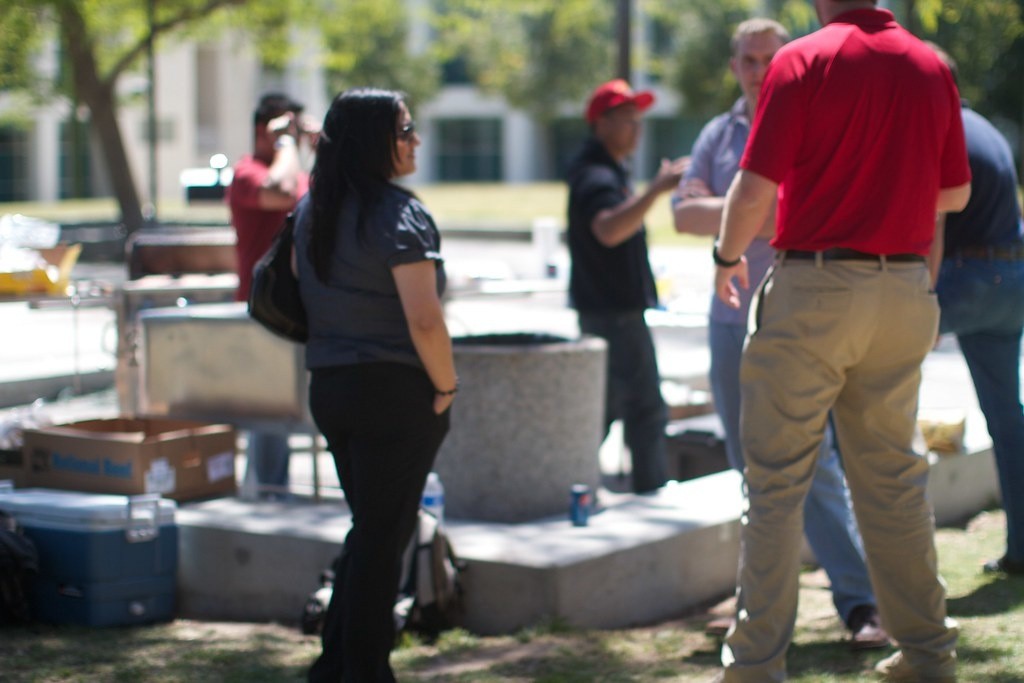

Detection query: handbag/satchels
xmin=247 ymin=211 xmax=310 ymax=343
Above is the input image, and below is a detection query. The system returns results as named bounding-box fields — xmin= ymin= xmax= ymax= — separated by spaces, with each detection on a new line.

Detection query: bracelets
xmin=432 ymin=377 xmax=459 ymax=395
xmin=713 ymin=246 xmax=742 ymax=266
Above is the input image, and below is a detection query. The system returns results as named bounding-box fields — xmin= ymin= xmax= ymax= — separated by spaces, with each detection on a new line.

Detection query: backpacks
xmin=304 ymin=514 xmax=474 ymax=644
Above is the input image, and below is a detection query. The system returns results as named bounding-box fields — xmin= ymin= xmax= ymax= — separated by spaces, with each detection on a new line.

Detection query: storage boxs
xmin=1 ymin=477 xmax=185 ymax=630
xmin=20 ymin=413 xmax=236 ymax=499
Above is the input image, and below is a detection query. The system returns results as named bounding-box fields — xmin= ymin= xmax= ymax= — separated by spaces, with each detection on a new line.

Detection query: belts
xmin=776 ymin=249 xmax=926 ymax=261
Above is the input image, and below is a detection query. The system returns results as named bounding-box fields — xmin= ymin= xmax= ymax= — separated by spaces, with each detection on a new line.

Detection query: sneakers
xmin=851 ymin=611 xmax=889 ymax=646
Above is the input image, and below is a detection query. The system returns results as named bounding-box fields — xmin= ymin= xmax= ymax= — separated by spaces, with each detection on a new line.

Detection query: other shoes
xmin=983 ymin=561 xmax=1005 ymax=573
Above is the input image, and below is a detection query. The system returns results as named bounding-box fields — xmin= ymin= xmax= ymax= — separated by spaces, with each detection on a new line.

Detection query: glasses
xmin=394 ymin=124 xmax=414 ymax=138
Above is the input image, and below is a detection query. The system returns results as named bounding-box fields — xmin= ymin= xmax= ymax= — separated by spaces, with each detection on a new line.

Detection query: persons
xmin=568 ymin=77 xmax=690 ymax=493
xmin=672 ymin=18 xmax=900 ymax=655
xmin=712 ymin=0 xmax=974 ymax=552
xmin=289 ymin=88 xmax=456 ymax=678
xmin=924 ymin=40 xmax=1023 ymax=573
xmin=229 ymin=94 xmax=322 ymax=502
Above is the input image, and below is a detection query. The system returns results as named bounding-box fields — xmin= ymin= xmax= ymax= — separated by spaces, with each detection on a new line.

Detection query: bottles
xmin=419 ymin=472 xmax=445 ymax=525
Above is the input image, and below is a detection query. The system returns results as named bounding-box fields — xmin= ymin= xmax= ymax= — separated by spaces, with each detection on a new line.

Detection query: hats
xmin=587 ymin=79 xmax=653 ymax=123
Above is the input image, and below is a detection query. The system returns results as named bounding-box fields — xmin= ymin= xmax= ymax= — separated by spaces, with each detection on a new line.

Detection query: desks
xmin=130 ymin=302 xmax=340 ymax=502
xmin=426 ymin=334 xmax=610 ymax=526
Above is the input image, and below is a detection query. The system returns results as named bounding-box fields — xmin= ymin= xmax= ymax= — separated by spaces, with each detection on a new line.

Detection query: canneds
xmin=571 ymin=484 xmax=590 ymax=525
